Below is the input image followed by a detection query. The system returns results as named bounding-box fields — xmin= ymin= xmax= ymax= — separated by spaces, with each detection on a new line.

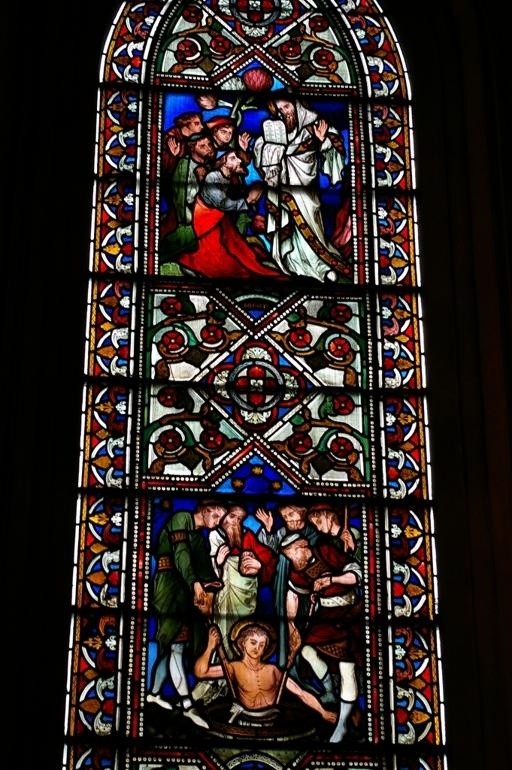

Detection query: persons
xmin=160 ymin=91 xmax=345 ymax=283
